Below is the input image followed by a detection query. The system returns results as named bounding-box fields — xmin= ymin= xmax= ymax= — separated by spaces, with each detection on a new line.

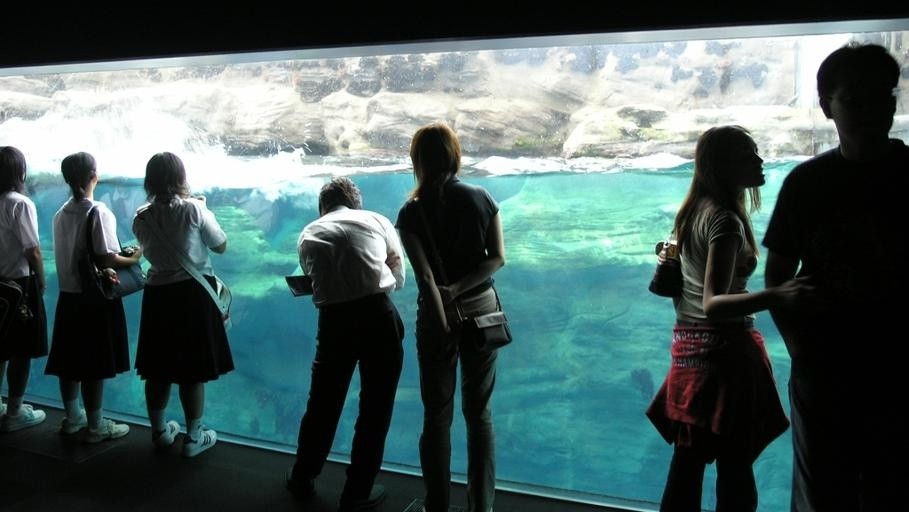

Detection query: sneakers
xmin=62 ymin=412 xmax=88 ymax=436
xmin=2 ymin=408 xmax=45 ymax=433
xmin=181 ymin=428 xmax=216 ymax=459
xmin=284 ymin=464 xmax=317 ymax=504
xmin=151 ymin=419 xmax=180 ymax=451
xmin=339 ymin=484 xmax=384 ymax=510
xmin=85 ymin=423 xmax=129 ymax=443
xmin=0 ymin=403 xmax=32 ymax=422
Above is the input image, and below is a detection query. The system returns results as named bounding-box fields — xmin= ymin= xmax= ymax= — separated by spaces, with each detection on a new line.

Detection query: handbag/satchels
xmin=78 ymin=245 xmax=146 ymax=304
xmin=0 ymin=277 xmax=36 ymax=349
xmin=649 ymin=240 xmax=683 ymax=299
xmin=213 ymin=274 xmax=232 ymax=318
xmin=452 ymin=311 xmax=512 ymax=354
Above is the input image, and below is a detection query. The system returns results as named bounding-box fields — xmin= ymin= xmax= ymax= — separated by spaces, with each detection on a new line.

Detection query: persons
xmin=133 ymin=151 xmax=235 ymax=458
xmin=286 ymin=176 xmax=405 ymax=511
xmin=660 ymin=126 xmax=817 ymax=511
xmin=394 ymin=124 xmax=506 ymax=511
xmin=0 ymin=145 xmax=48 ymax=433
xmin=52 ymin=152 xmax=144 ymax=443
xmin=765 ymin=44 xmax=907 ymax=511
